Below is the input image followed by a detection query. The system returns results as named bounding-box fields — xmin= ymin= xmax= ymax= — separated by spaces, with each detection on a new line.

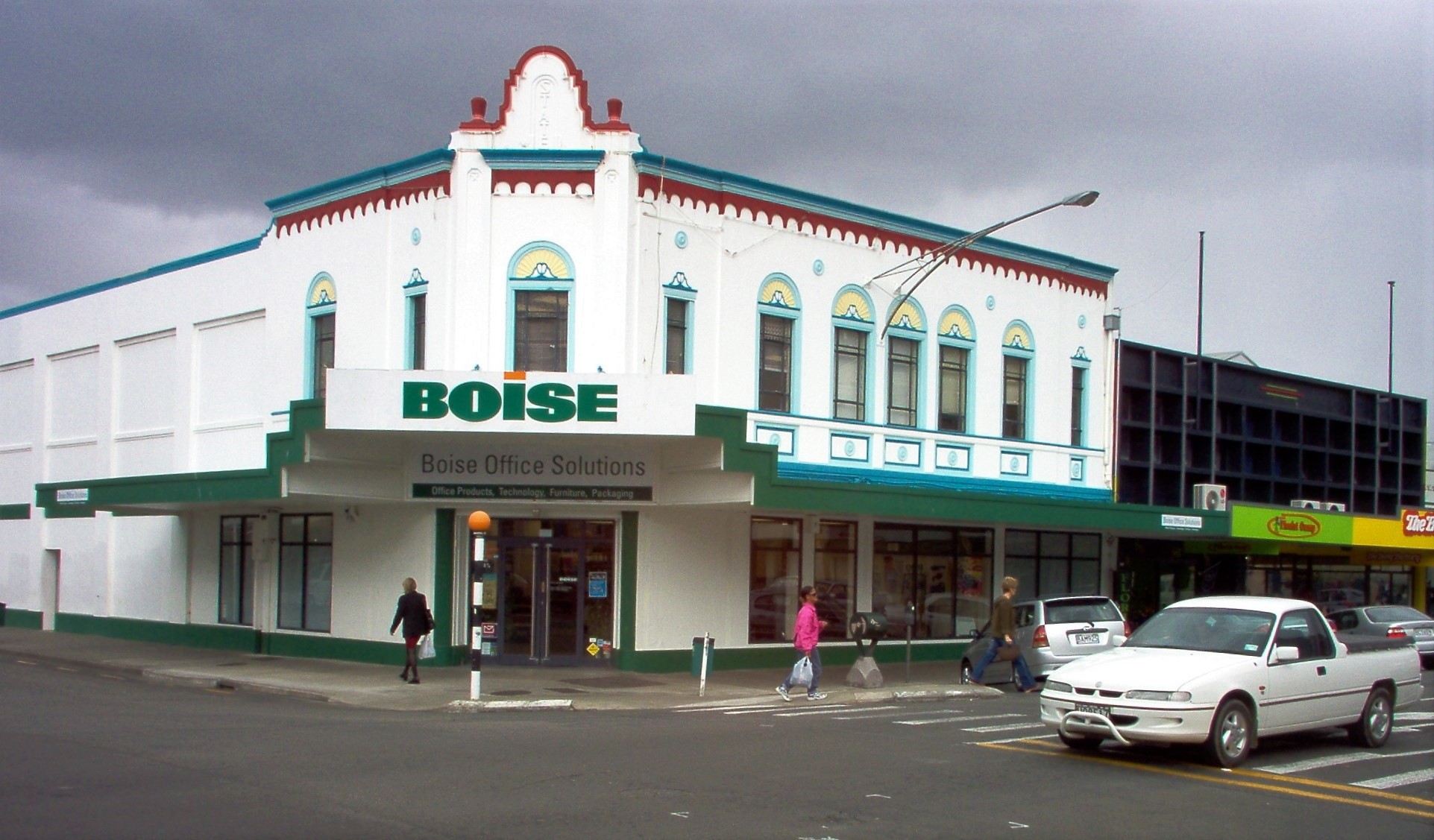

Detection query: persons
xmin=390 ymin=578 xmax=430 ymax=684
xmin=969 ymin=577 xmax=1041 ymax=693
xmin=775 ymin=586 xmax=828 ymax=701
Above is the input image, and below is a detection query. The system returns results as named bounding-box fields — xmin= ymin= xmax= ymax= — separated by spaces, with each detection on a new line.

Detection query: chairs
xmin=1189 ymin=615 xmax=1311 ymax=655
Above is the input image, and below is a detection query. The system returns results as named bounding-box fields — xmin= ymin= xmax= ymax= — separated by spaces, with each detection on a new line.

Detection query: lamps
xmin=873 ymin=190 xmax=1101 ymax=340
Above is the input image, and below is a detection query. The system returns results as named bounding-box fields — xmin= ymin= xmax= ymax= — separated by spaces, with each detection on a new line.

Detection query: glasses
xmin=809 ymin=592 xmax=818 ymax=596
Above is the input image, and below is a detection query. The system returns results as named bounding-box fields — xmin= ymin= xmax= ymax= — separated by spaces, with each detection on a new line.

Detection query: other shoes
xmin=400 ymin=672 xmax=408 ymax=680
xmin=408 ymin=679 xmax=421 ymax=684
xmin=968 ymin=678 xmax=985 ymax=687
xmin=1025 ymin=681 xmax=1045 ymax=693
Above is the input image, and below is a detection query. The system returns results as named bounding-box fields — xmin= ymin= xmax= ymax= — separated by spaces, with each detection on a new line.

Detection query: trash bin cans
xmin=692 ymin=637 xmax=717 ymax=677
xmin=0 ymin=602 xmax=7 ymax=628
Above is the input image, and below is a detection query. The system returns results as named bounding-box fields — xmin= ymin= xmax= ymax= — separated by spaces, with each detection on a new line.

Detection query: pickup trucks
xmin=1038 ymin=594 xmax=1426 ymax=768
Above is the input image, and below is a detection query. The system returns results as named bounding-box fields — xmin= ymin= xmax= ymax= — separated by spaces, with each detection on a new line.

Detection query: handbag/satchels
xmin=418 ymin=635 xmax=436 ymax=660
xmin=790 ymin=656 xmax=813 ymax=689
xmin=997 ymin=643 xmax=1020 ymax=661
xmin=422 ymin=594 xmax=436 ymax=630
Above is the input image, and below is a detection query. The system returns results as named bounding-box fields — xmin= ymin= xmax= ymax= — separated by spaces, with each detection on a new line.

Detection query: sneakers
xmin=807 ymin=691 xmax=827 ymax=700
xmin=776 ymin=683 xmax=790 ymax=701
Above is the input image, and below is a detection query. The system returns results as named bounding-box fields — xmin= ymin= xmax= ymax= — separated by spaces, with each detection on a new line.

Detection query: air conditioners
xmin=1290 ymin=500 xmax=1345 ymax=511
xmin=1193 ymin=484 xmax=1226 ymax=510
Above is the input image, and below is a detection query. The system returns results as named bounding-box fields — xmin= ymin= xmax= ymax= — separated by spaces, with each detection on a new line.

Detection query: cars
xmin=751 ymin=570 xmax=993 ymax=635
xmin=1310 ymin=586 xmax=1363 ymax=613
xmin=957 ymin=592 xmax=1136 ymax=692
xmin=1327 ymin=605 xmax=1434 ymax=670
